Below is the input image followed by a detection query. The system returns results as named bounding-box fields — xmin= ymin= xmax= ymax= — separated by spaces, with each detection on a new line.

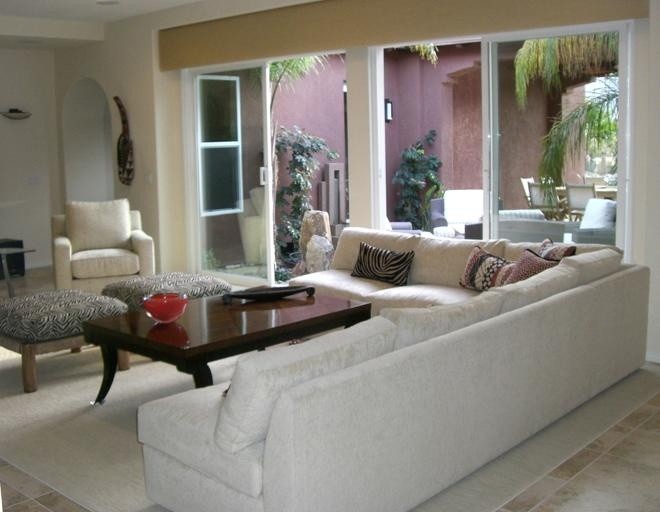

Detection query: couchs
xmin=289 ymin=226 xmax=625 ymax=311
xmin=137 ymin=262 xmax=650 ymax=512
xmin=464 ymin=219 xmax=615 ymax=251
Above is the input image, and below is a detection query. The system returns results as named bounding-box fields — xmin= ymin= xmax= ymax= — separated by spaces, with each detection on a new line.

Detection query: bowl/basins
xmin=140 ymin=292 xmax=188 ymax=325
xmin=146 ymin=323 xmax=190 ymax=349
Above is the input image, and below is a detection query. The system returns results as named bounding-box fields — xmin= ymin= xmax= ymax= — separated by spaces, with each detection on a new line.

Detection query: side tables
xmin=0 ymin=247 xmax=35 ymax=297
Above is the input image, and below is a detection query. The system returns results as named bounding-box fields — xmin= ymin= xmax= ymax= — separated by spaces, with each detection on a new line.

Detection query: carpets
xmin=0 ymin=326 xmax=660 ymax=511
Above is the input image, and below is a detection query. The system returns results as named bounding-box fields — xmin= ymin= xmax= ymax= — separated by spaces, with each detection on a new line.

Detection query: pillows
xmin=331 ymin=228 xmax=415 ymax=277
xmin=459 ymin=245 xmax=515 ymax=291
xmin=214 ymin=314 xmax=395 ymax=453
xmin=64 ymin=198 xmax=131 ymax=253
xmin=351 ymin=242 xmax=414 ymax=285
xmin=381 ymin=291 xmax=504 ymax=351
xmin=504 ymin=249 xmax=559 ymax=285
xmin=489 ymin=264 xmax=580 ymax=317
xmin=411 ymin=237 xmax=507 ymax=290
xmin=579 ymin=198 xmax=616 ymax=230
xmin=560 ymin=249 xmax=622 ymax=286
xmin=508 ymin=242 xmax=624 ymax=263
xmin=444 ymin=189 xmax=483 ymax=234
xmin=535 ymin=238 xmax=576 ymax=260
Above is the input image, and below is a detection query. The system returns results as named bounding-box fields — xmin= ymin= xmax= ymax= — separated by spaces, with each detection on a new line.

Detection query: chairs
xmin=500 ymin=208 xmax=545 ymax=221
xmin=430 ymin=197 xmax=502 ymax=227
xmin=519 ymin=175 xmax=595 ymax=222
xmin=51 ymin=210 xmax=156 ymax=292
xmin=389 ymin=222 xmax=420 ymax=236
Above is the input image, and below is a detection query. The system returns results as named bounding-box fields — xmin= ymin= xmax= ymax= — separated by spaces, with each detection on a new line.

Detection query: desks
xmin=594 ymin=184 xmax=617 ymax=200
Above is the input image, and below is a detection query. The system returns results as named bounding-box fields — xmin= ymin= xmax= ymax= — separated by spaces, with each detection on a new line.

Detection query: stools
xmin=102 ymin=271 xmax=232 ymax=310
xmin=433 ymin=225 xmax=455 ymax=238
xmin=0 ymin=287 xmax=130 ymax=393
